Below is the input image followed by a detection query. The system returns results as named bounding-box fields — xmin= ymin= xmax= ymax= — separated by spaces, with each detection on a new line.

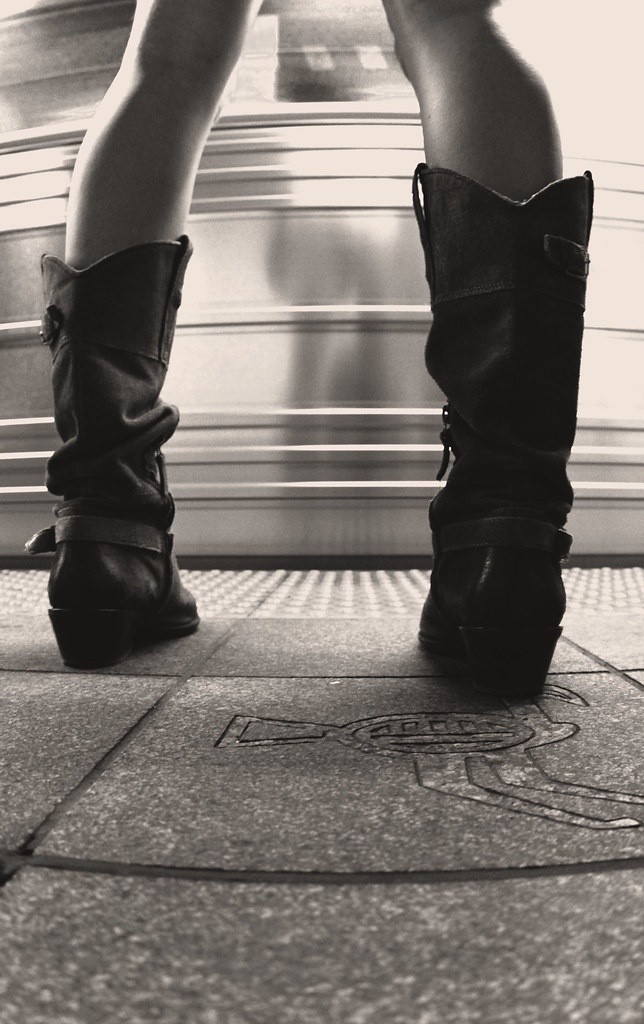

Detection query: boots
xmin=27 ymin=232 xmax=202 ymax=669
xmin=411 ymin=160 xmax=596 ymax=702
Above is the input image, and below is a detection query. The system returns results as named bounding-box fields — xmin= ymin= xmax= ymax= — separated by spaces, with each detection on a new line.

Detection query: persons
xmin=37 ymin=0 xmax=593 ymax=698
